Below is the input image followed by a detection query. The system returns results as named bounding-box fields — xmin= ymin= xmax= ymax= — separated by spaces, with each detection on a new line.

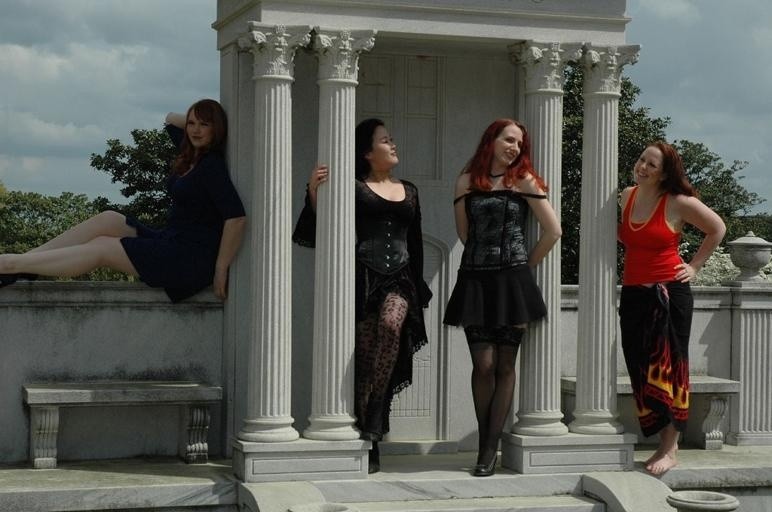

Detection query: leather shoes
xmin=474 ymin=452 xmax=497 ymax=476
xmin=369 ymin=450 xmax=379 ymax=472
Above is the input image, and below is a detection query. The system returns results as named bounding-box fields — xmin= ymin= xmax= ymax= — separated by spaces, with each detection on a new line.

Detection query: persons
xmin=0 ymin=99 xmax=247 ymax=305
xmin=616 ymin=141 xmax=727 ymax=477
xmin=442 ymin=119 xmax=563 ymax=475
xmin=291 ymin=117 xmax=433 ymax=474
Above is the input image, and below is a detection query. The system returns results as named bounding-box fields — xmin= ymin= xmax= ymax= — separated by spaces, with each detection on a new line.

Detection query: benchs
xmin=561 ymin=376 xmax=741 ymax=450
xmin=23 ymin=380 xmax=223 ymax=469
